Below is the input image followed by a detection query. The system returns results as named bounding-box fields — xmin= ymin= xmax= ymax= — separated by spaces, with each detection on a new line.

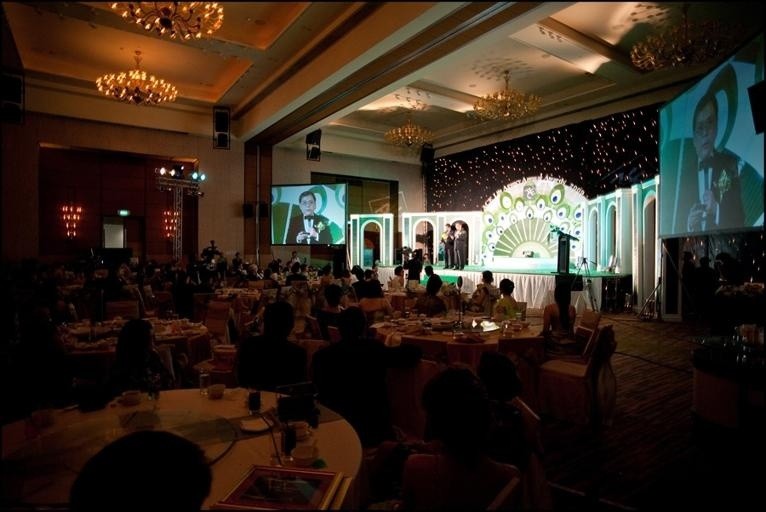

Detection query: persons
xmin=395 ymin=363 xmax=525 ymax=512
xmin=478 ymin=352 xmax=524 ymax=402
xmin=677 ymin=91 xmax=746 ymax=233
xmin=424 ymin=264 xmax=443 ymax=297
xmin=0 ymin=251 xmax=77 ymax=409
xmin=540 ymin=281 xmax=581 ymax=351
xmin=311 ymin=305 xmax=344 ymax=339
xmin=439 ymin=222 xmax=455 ymax=269
xmin=470 ymin=270 xmax=497 ymax=314
xmin=77 ymin=315 xmax=170 ymax=414
xmin=492 ymin=278 xmax=525 ymax=318
xmin=402 ymin=247 xmax=423 ymax=284
xmin=682 ymin=248 xmax=764 ymax=326
xmin=387 ymin=266 xmax=404 ymax=289
xmin=67 ymin=431 xmax=213 ymax=512
xmin=453 ymin=222 xmax=467 ymax=271
xmin=234 ymin=299 xmax=308 ymax=395
xmin=311 ymin=304 xmax=390 ymax=444
xmin=285 ymin=191 xmax=332 ymax=245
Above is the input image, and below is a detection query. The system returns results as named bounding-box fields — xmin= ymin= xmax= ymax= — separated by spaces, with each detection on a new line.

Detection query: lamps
xmin=96 ymin=50 xmax=182 ymax=105
xmin=629 ymin=4 xmax=750 ymax=71
xmin=383 ymin=111 xmax=439 ymax=148
xmin=108 ymin=3 xmax=225 ymax=41
xmin=156 ymin=166 xmax=212 ymax=183
xmin=474 ymin=69 xmax=541 ymax=122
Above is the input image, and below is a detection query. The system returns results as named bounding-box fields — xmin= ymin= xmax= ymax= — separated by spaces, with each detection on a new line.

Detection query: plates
xmin=291 ymin=446 xmax=319 ymax=467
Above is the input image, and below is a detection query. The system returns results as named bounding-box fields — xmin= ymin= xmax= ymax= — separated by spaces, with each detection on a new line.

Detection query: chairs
xmin=539 ymin=324 xmax=614 ymax=427
xmin=361 ymin=284 xmax=530 ymax=316
xmin=208 ymin=280 xmax=323 ymax=323
xmin=546 ymin=306 xmax=602 ymax=365
xmin=53 ymin=262 xmax=208 ymax=317
xmin=207 ymin=324 xmax=372 ymax=384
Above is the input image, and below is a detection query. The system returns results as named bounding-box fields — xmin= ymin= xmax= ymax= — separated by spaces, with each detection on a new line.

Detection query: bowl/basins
xmin=513 ymin=323 xmax=523 ymax=330
xmin=519 ymin=321 xmax=531 ymax=327
xmin=121 ymin=391 xmax=140 ymax=401
xmin=208 ymin=384 xmax=225 ymax=399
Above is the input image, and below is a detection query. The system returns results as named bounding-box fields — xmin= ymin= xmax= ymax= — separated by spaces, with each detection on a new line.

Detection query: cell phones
xmin=301 ymin=232 xmax=311 ymax=235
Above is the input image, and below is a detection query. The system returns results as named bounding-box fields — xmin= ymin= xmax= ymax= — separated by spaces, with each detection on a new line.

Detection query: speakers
xmin=211 ymin=104 xmax=232 ymax=149
xmin=306 ymin=128 xmax=321 ymax=162
xmin=243 ymin=202 xmax=253 ymax=218
xmin=419 ymin=141 xmax=436 ymax=177
xmin=259 ymin=202 xmax=268 ymax=217
xmin=747 ymin=79 xmax=766 ymax=136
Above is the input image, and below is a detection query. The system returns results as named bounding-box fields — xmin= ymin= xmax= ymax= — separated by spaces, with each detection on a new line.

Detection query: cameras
xmin=587 ymin=279 xmax=592 ymax=283
xmin=399 ymin=245 xmax=413 ymax=254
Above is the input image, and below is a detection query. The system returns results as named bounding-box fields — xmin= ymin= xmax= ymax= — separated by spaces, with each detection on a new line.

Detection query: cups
xmin=392 ymin=319 xmax=400 ymax=332
xmin=502 ymin=322 xmax=509 ymax=336
xmin=248 ymin=390 xmax=262 ymax=414
xmin=516 ymin=312 xmax=522 ymax=324
xmin=384 ymin=315 xmax=392 ymax=328
xmin=199 ymin=367 xmax=209 ymax=396
xmin=280 ymin=424 xmax=297 ymax=461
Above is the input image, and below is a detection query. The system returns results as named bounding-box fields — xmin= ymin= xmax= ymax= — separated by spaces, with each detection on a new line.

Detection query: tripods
xmin=571 ymin=265 xmax=593 ymax=290
xmin=577 ymin=284 xmax=600 ymax=314
